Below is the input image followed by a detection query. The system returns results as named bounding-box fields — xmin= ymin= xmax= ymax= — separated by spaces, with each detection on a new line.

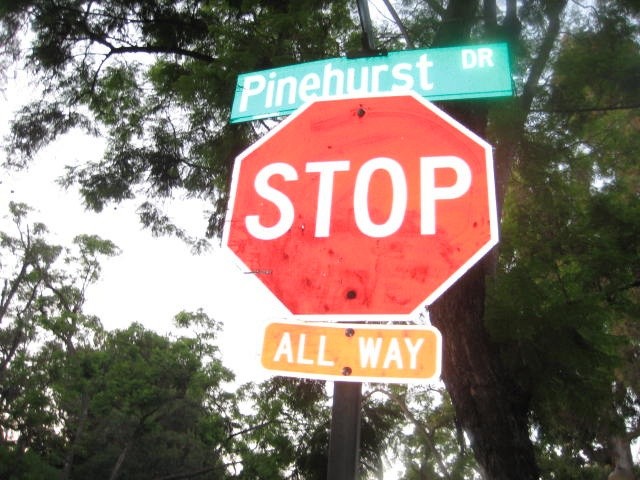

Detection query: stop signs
xmin=220 ymin=90 xmax=500 ymax=322
